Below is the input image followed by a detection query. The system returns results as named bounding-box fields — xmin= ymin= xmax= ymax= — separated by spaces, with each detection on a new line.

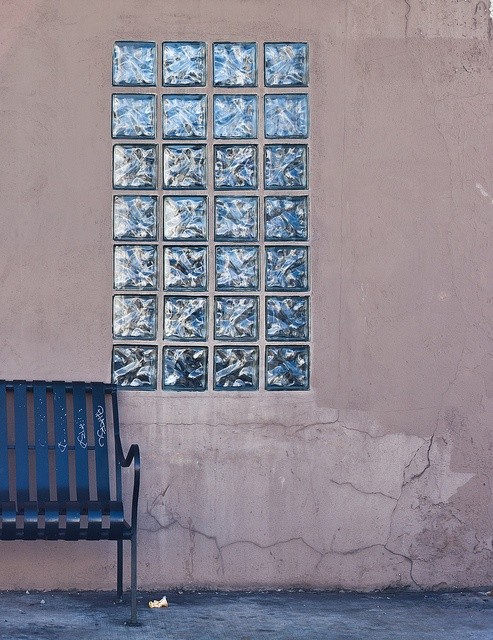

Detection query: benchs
xmin=0 ymin=379 xmax=148 ymax=629
